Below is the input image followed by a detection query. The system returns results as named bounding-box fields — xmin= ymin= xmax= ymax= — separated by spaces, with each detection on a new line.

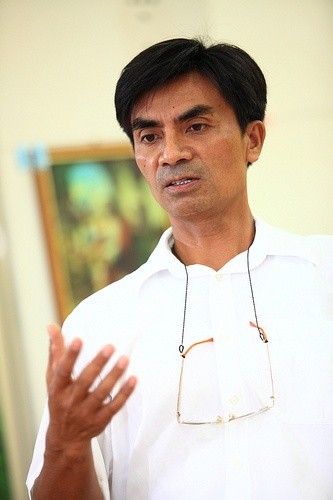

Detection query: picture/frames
xmin=27 ymin=142 xmax=171 ymax=330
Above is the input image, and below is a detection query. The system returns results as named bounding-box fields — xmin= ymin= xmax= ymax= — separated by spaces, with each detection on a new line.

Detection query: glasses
xmin=176 ymin=321 xmax=275 ymax=427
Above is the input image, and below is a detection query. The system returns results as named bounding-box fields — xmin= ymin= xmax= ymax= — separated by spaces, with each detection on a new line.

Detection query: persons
xmin=23 ymin=36 xmax=332 ymax=500
